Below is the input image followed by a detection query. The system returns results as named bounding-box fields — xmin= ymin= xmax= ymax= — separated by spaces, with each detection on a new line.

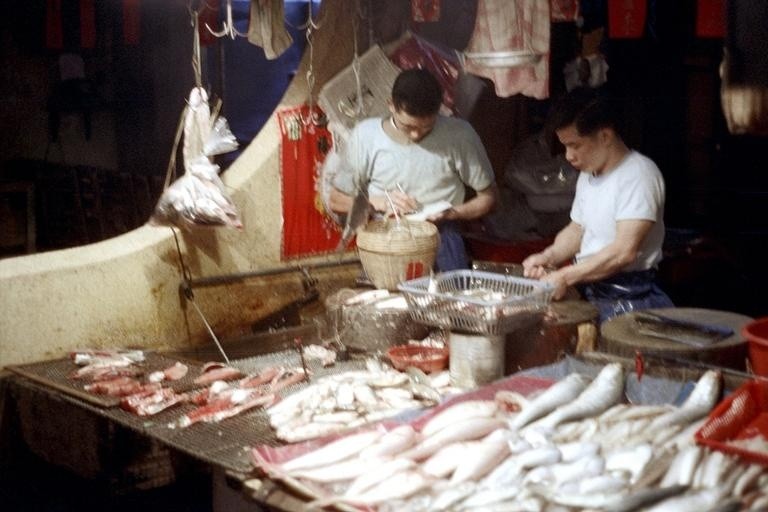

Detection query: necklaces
xmin=389 ymin=114 xmax=400 ymax=132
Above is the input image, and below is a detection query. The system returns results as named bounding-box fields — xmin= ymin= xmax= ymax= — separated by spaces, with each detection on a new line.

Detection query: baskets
xmin=694 ymin=380 xmax=767 ymax=464
xmin=396 ymin=268 xmax=555 ymax=337
xmin=355 ymin=209 xmax=439 ymax=291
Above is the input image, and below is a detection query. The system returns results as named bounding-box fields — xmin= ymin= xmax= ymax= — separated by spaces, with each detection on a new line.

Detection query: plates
xmin=464 ymin=48 xmax=544 ymax=72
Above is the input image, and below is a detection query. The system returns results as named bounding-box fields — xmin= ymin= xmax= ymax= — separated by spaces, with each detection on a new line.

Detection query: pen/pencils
xmin=397 ymin=183 xmax=417 ymax=214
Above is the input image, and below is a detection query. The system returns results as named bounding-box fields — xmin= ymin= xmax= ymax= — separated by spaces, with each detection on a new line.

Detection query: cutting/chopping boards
xmin=321 ymin=286 xmax=439 ymax=359
xmin=599 ymin=305 xmax=761 ymax=383
xmin=497 ymin=300 xmax=603 ymax=379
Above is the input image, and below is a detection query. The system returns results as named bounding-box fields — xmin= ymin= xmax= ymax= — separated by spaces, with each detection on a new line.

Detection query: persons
xmin=522 ymin=84 xmax=677 ymax=322
xmin=320 ymin=67 xmax=497 ymax=289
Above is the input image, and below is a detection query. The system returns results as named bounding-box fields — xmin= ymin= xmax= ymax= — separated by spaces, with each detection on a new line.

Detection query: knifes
xmin=466 ymin=261 xmax=560 ymax=296
xmin=629 ymin=307 xmax=736 ymax=349
